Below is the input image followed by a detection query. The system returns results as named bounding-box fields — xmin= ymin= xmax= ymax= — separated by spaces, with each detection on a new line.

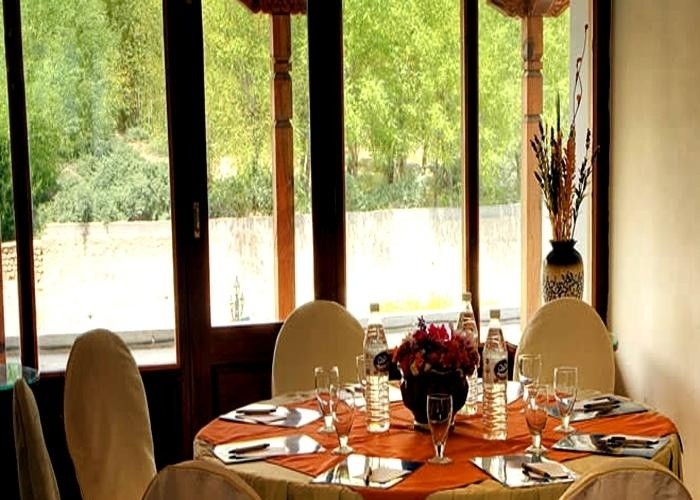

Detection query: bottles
xmin=455 ymin=292 xmax=480 ymax=416
xmin=483 ymin=309 xmax=508 ymax=440
xmin=366 ymin=303 xmax=390 ymax=433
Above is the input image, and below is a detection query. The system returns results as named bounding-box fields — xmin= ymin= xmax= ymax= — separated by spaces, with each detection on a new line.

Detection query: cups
xmin=355 ymin=355 xmax=367 ymax=399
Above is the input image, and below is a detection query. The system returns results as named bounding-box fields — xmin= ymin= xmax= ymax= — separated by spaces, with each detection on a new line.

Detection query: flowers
xmin=384 ymin=314 xmax=482 ymax=379
xmin=531 ymin=24 xmax=604 ymax=239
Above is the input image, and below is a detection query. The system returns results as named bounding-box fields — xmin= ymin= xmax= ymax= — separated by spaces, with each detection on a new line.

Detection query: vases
xmin=396 ymin=371 xmax=470 ymax=428
xmin=544 ymin=238 xmax=585 ymax=299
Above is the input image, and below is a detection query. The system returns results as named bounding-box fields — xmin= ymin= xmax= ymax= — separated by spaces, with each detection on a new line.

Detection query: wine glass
xmin=518 ymin=352 xmax=547 ymax=454
xmin=426 ymin=393 xmax=454 ymax=464
xmin=314 ymin=364 xmax=355 ymax=455
xmin=552 ymin=367 xmax=578 ymax=434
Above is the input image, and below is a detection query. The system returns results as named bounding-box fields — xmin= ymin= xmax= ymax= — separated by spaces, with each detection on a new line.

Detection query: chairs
xmin=10 ymin=377 xmax=64 ymax=498
xmin=557 ymin=458 xmax=692 ymax=499
xmin=60 ymin=328 xmax=159 ymax=499
xmin=511 ymin=297 xmax=615 ymax=396
xmin=268 ymin=299 xmax=370 ymax=396
xmin=139 ymin=458 xmax=254 ymax=498
